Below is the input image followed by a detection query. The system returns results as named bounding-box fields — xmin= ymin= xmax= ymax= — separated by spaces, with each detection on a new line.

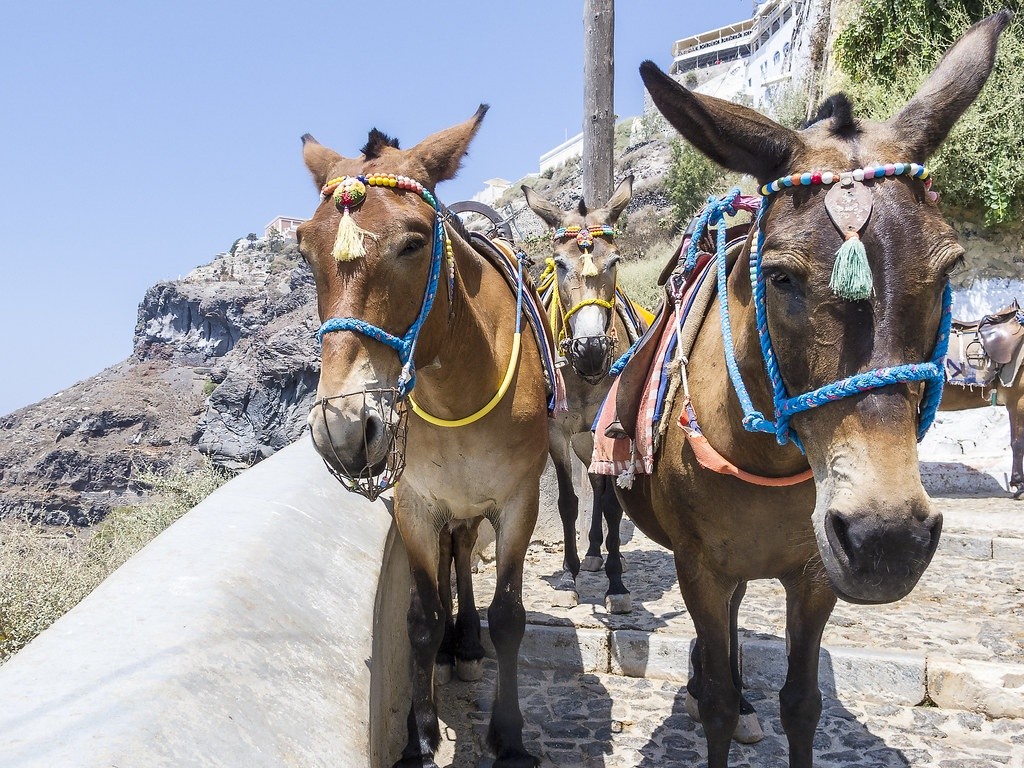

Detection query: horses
xmin=294 ymin=102 xmax=556 ymax=767
xmin=514 ymin=174 xmax=656 ymax=616
xmin=935 ymin=295 xmax=1024 ymax=502
xmin=610 ymin=7 xmax=1013 ymax=768
